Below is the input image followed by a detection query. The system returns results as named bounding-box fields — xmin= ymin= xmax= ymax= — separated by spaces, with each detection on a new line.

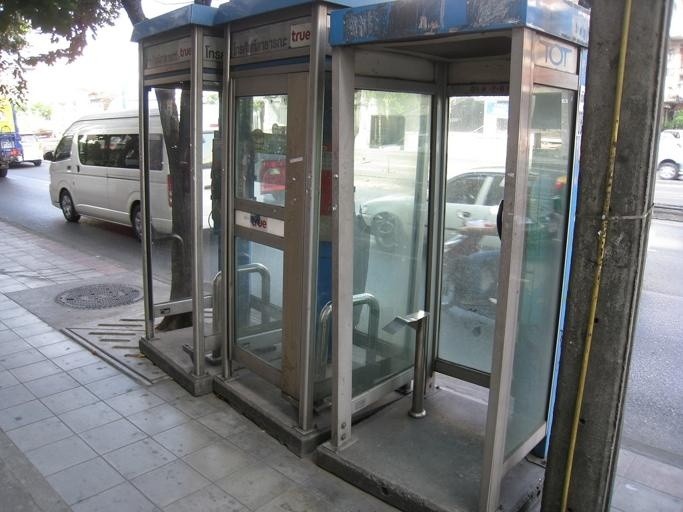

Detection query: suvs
xmin=9 ymin=132 xmax=40 ymax=169
xmin=655 ymin=128 xmax=683 ymax=178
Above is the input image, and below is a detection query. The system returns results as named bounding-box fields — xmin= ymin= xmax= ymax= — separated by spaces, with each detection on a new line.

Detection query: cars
xmin=471 ymin=123 xmax=564 ymax=150
xmin=360 ymin=167 xmax=506 ymax=252
xmin=34 ymin=130 xmax=56 ymax=138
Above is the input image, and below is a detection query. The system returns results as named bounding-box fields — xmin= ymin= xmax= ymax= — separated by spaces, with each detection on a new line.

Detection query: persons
xmin=458 ymin=192 xmax=550 ymax=308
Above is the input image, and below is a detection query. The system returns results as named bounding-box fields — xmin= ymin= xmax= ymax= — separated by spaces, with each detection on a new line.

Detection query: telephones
xmin=496 ymin=196 xmax=504 ymax=241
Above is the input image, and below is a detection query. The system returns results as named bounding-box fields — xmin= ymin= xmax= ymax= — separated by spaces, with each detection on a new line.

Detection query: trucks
xmin=0 ymin=125 xmax=24 ymax=178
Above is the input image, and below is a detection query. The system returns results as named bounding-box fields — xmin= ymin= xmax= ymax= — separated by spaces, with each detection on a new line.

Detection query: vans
xmin=42 ymin=105 xmax=219 ymax=245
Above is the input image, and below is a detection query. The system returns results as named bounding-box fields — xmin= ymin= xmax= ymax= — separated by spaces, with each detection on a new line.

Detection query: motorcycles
xmin=441 ymin=206 xmax=560 ymax=335
